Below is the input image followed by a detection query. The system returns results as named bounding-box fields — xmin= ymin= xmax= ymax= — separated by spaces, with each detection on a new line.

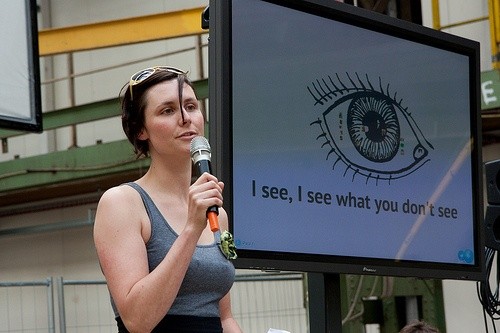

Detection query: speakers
xmin=484 ymin=205 xmax=500 ymax=250
xmin=485 ymin=159 xmax=500 ymax=205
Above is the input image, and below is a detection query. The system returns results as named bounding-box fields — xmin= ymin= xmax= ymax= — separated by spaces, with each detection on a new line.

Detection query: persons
xmin=92 ymin=64 xmax=235 ymax=333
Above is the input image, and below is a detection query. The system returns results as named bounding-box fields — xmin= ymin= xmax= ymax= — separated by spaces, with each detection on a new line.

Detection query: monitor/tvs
xmin=209 ymin=0 xmax=486 ymax=286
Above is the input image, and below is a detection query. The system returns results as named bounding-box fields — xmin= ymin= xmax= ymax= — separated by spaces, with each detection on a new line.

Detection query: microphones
xmin=190 ymin=136 xmax=219 ymax=232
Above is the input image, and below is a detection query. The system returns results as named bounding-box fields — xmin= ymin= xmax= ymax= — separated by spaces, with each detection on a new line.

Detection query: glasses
xmin=129 ymin=67 xmax=185 ymax=101
